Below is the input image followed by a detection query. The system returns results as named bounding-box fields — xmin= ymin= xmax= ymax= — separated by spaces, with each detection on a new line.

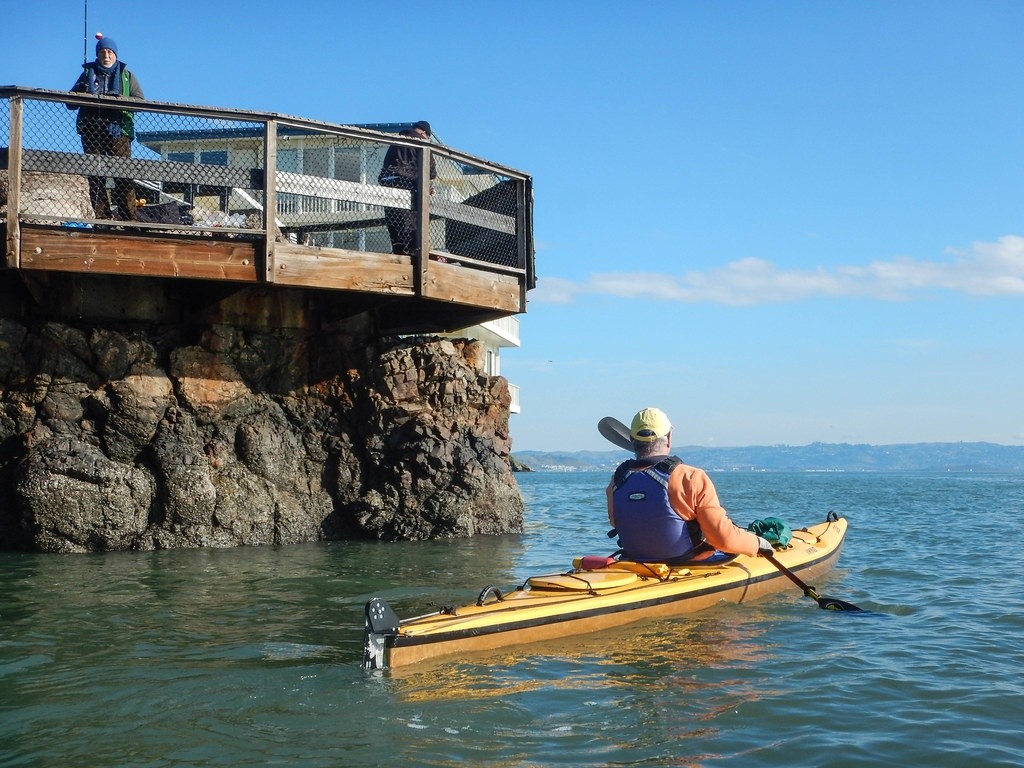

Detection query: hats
xmin=96 ymin=38 xmax=117 ymax=58
xmin=414 ymin=121 xmax=431 ymax=136
xmin=630 ymin=407 xmax=675 ymax=442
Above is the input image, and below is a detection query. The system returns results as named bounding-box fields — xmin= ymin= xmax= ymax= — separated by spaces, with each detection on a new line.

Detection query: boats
xmin=360 ymin=509 xmax=848 ymax=670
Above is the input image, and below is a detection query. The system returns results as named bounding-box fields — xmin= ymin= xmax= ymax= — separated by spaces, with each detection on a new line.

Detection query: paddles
xmin=597 ymin=415 xmax=864 ymax=611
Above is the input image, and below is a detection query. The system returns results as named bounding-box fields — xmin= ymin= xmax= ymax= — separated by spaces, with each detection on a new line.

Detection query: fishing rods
xmin=83 ymin=0 xmax=89 ymax=93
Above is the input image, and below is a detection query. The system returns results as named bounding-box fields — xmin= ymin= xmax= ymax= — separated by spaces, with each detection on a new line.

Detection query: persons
xmin=378 ymin=121 xmax=437 ymax=259
xmin=67 ymin=37 xmax=143 ymax=222
xmin=607 ymin=408 xmax=771 ymax=564
